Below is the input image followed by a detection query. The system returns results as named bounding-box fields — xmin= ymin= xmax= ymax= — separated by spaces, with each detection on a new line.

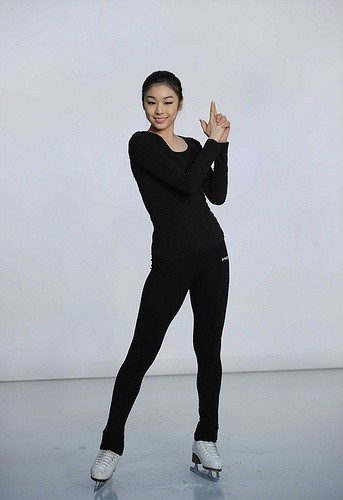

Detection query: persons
xmin=89 ymin=71 xmax=230 ymax=491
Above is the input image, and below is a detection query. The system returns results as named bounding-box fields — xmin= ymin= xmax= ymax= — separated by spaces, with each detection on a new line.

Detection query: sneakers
xmin=90 ymin=449 xmax=120 ymax=492
xmin=189 ymin=440 xmax=223 ymax=482
